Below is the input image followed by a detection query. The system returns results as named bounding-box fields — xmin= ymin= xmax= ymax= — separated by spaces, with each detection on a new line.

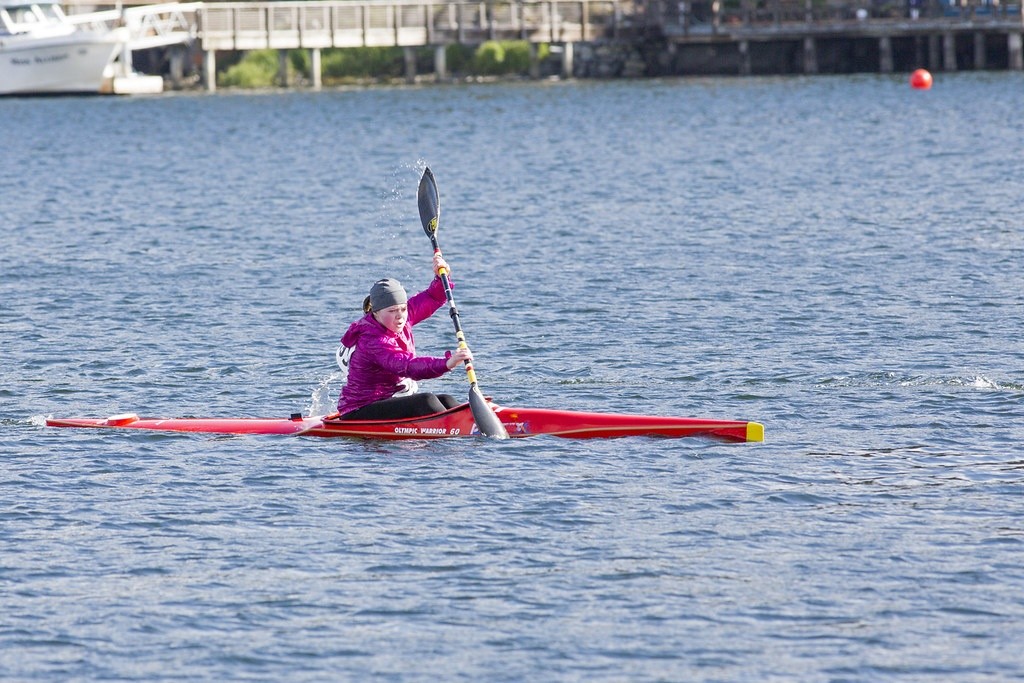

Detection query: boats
xmin=0 ymin=0 xmax=218 ymax=98
xmin=44 ymin=396 xmax=766 ymax=443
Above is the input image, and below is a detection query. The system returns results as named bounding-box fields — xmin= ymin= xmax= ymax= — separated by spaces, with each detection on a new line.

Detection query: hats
xmin=370 ymin=278 xmax=408 ymax=311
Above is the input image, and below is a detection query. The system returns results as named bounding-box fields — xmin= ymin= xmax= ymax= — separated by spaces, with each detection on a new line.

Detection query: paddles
xmin=416 ymin=167 xmax=510 ymax=441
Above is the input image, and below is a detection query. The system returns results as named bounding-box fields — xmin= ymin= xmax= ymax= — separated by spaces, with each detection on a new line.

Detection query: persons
xmin=337 ymin=255 xmax=473 ymax=420
